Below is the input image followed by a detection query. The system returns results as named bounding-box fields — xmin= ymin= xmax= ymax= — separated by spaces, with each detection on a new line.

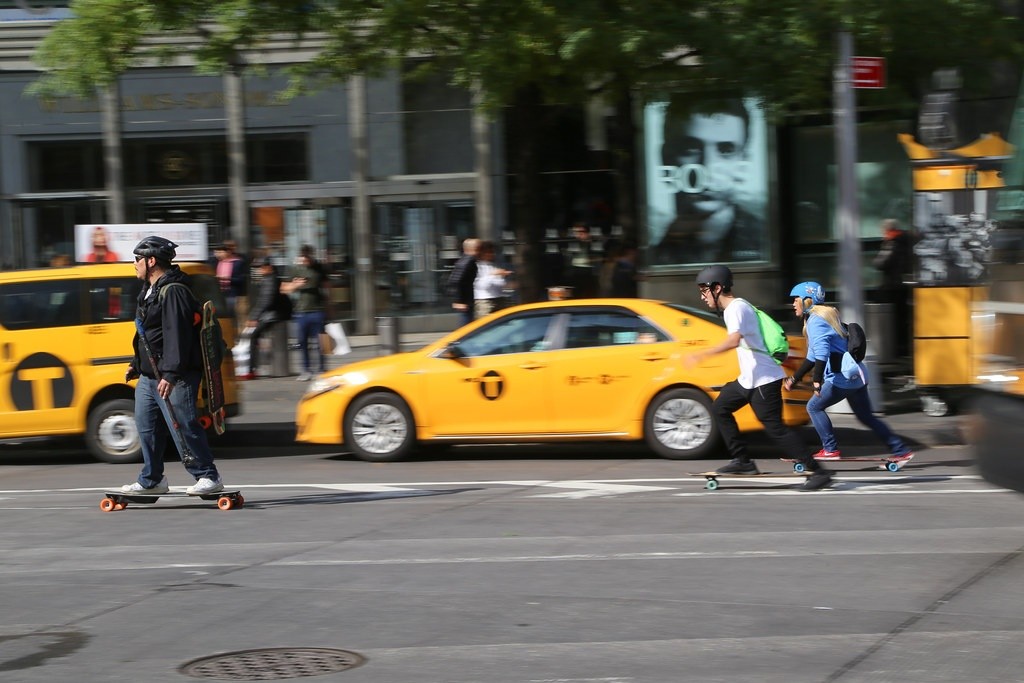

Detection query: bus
xmin=894 ymin=129 xmax=1024 ymax=490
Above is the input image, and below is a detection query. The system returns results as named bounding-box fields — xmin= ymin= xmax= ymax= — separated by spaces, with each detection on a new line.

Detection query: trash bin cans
xmin=860 ymin=301 xmax=896 ymax=414
xmin=375 ymin=316 xmax=400 ymax=357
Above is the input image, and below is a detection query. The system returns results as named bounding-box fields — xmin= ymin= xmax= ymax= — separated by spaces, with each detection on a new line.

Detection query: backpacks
xmin=734 ymin=295 xmax=789 ymax=365
xmin=847 ymin=322 xmax=867 ymax=364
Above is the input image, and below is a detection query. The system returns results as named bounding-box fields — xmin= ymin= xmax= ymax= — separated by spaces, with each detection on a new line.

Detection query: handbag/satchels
xmin=319 ymin=330 xmax=333 ymax=354
xmin=324 ymin=321 xmax=352 ymax=355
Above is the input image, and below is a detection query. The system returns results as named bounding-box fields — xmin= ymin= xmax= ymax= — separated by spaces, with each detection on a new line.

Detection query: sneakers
xmin=186 ymin=478 xmax=224 ymax=495
xmin=879 ymin=451 xmax=915 ymax=470
xmin=812 ymin=449 xmax=841 ymax=460
xmin=715 ymin=457 xmax=759 ymax=475
xmin=799 ymin=471 xmax=835 ymax=491
xmin=121 ymin=475 xmax=169 ymax=494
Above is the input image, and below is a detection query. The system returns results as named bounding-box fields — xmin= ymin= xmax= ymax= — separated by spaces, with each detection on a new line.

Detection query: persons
xmin=446 ymin=238 xmax=515 ymax=326
xmin=88 ymin=226 xmax=116 ymax=263
xmin=211 ymin=241 xmax=330 ymax=381
xmin=542 ymin=240 xmax=638 ymax=299
xmin=649 ymin=99 xmax=766 ymax=263
xmin=121 ymin=236 xmax=224 ymax=494
xmin=783 ymin=282 xmax=913 ymax=460
xmin=45 ymin=246 xmax=75 ymax=267
xmin=688 ymin=263 xmax=818 ymax=474
xmin=876 ymin=219 xmax=905 ymax=289
xmin=373 ymin=252 xmax=396 ymax=308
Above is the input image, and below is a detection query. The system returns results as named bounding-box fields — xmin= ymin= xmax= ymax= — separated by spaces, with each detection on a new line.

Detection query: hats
xmin=249 ymin=255 xmax=270 ymax=267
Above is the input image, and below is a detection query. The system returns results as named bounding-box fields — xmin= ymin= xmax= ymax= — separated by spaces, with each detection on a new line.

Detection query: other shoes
xmin=296 ymin=371 xmax=324 ymax=381
xmin=235 ymin=373 xmax=256 ymax=380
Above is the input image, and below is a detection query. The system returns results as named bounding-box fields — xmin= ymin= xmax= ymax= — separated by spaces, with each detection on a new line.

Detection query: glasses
xmin=700 ymin=288 xmax=710 ymax=296
xmin=135 ymin=256 xmax=145 ymax=262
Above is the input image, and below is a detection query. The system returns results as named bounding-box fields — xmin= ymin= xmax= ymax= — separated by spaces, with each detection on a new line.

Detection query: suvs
xmin=1 ymin=262 xmax=244 ymax=466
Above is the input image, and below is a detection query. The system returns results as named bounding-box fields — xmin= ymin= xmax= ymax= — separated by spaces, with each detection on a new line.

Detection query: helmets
xmin=696 ymin=265 xmax=734 ymax=293
xmin=789 ymin=281 xmax=825 ymax=305
xmin=133 ymin=236 xmax=179 ymax=262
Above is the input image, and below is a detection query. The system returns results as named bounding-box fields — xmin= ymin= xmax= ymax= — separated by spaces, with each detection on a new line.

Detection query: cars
xmin=291 ymin=293 xmax=814 ymax=463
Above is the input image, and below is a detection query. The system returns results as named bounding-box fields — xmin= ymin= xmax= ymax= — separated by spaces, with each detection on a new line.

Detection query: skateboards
xmin=99 ymin=491 xmax=244 ymax=511
xmin=193 ymin=300 xmax=226 ymax=436
xmin=687 ymin=471 xmax=818 ymax=490
xmin=781 ymin=452 xmax=914 ymax=471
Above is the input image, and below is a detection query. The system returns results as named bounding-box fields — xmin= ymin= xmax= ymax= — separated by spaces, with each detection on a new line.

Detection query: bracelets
xmin=790 ymin=377 xmax=796 ymax=383
